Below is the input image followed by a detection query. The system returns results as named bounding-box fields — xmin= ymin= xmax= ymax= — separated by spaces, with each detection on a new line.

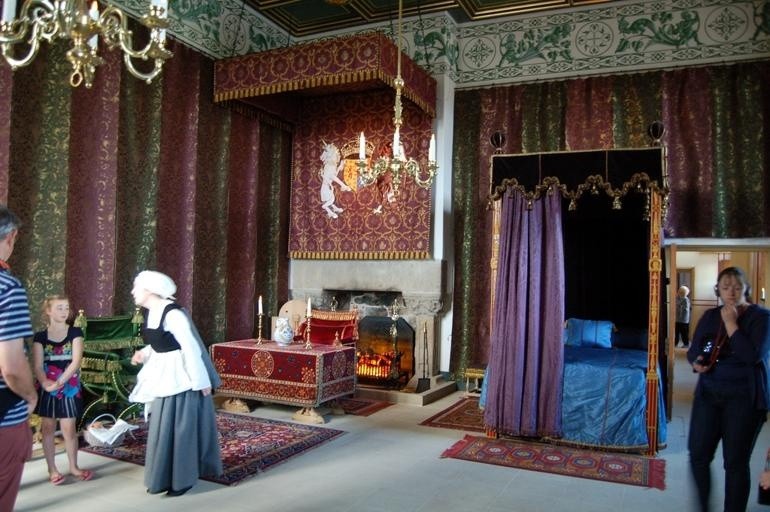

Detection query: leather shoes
xmin=166 ymin=486 xmax=192 ymax=497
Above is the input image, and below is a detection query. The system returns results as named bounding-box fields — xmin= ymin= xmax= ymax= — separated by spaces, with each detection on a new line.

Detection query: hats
xmin=133 ymin=270 xmax=178 ymax=300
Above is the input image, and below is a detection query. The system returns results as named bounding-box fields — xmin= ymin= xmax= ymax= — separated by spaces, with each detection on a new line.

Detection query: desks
xmin=209 ymin=337 xmax=359 ymax=423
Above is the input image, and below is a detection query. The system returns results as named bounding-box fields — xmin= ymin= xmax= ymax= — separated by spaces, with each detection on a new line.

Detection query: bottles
xmin=73 ymin=309 xmax=87 ymax=341
xmin=130 ymin=308 xmax=145 ymax=340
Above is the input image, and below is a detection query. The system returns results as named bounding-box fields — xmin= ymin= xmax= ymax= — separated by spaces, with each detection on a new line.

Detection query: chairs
xmin=292 ymin=309 xmax=359 ymax=349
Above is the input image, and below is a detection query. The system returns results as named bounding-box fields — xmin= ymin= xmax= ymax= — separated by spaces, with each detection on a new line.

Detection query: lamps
xmin=0 ymin=0 xmax=177 ymax=89
xmin=352 ymin=0 xmax=438 ymax=198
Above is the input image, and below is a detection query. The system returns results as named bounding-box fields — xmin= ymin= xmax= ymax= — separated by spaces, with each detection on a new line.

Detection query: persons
xmin=674 ymin=266 xmax=770 ymax=512
xmin=0 ymin=206 xmax=39 ymax=512
xmin=33 ymin=293 xmax=96 ymax=486
xmin=128 ymin=270 xmax=224 ymax=496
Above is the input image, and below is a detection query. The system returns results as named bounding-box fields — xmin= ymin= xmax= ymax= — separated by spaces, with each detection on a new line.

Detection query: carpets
xmin=417 ymin=395 xmax=488 ymax=432
xmin=326 ymin=397 xmax=397 ymax=417
xmin=77 ymin=409 xmax=345 ymax=486
xmin=438 ymin=433 xmax=665 ymax=491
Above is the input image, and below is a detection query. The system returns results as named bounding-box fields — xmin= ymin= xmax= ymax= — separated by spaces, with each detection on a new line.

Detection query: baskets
xmin=83 ymin=414 xmax=124 ymax=448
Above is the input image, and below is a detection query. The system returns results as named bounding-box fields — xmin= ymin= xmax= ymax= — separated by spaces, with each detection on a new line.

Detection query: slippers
xmin=80 ymin=470 xmax=94 ymax=480
xmin=49 ymin=475 xmax=65 ymax=485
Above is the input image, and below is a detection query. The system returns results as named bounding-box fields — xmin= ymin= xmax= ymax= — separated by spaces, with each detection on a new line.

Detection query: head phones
xmin=714 ymin=285 xmax=752 ymax=297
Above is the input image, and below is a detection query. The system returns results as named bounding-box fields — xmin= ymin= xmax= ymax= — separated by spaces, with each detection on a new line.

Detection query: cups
xmin=333 ymin=339 xmax=342 ymax=351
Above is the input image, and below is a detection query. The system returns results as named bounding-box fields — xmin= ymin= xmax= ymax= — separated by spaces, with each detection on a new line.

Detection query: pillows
xmin=567 ymin=318 xmax=617 ymax=350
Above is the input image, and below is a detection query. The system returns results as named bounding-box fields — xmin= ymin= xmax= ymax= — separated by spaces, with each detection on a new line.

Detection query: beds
xmin=479 ymin=120 xmax=669 ymax=458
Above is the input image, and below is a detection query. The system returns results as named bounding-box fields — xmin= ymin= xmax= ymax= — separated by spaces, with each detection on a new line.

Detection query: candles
xmin=308 ymin=297 xmax=312 ymax=317
xmin=257 ymin=294 xmax=263 ymax=314
